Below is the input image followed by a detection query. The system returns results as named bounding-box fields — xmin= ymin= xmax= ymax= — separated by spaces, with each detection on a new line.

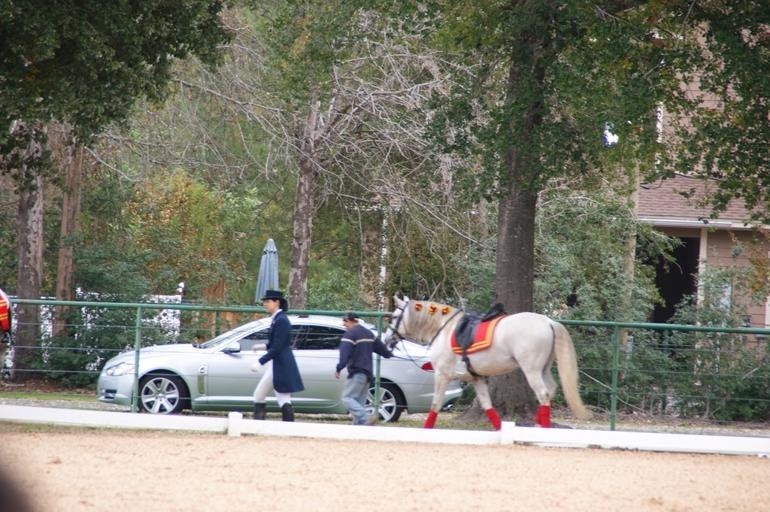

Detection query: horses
xmin=381 ymin=295 xmax=593 ymax=428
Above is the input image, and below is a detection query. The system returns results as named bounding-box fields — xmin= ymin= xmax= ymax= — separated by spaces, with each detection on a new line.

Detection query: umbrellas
xmin=254 ymin=237 xmax=280 ymax=305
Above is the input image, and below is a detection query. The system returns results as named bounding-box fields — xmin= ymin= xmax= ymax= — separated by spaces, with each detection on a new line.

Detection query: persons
xmin=252 ymin=290 xmax=306 ymax=421
xmin=335 ymin=310 xmax=392 ymax=425
xmin=0 ymin=288 xmax=13 ymax=372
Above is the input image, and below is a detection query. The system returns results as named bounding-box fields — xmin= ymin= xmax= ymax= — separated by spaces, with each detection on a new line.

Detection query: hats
xmin=261 ymin=290 xmax=282 ymax=300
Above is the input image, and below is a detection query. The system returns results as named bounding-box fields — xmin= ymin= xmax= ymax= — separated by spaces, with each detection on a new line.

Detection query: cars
xmin=91 ymin=308 xmax=465 ymax=425
